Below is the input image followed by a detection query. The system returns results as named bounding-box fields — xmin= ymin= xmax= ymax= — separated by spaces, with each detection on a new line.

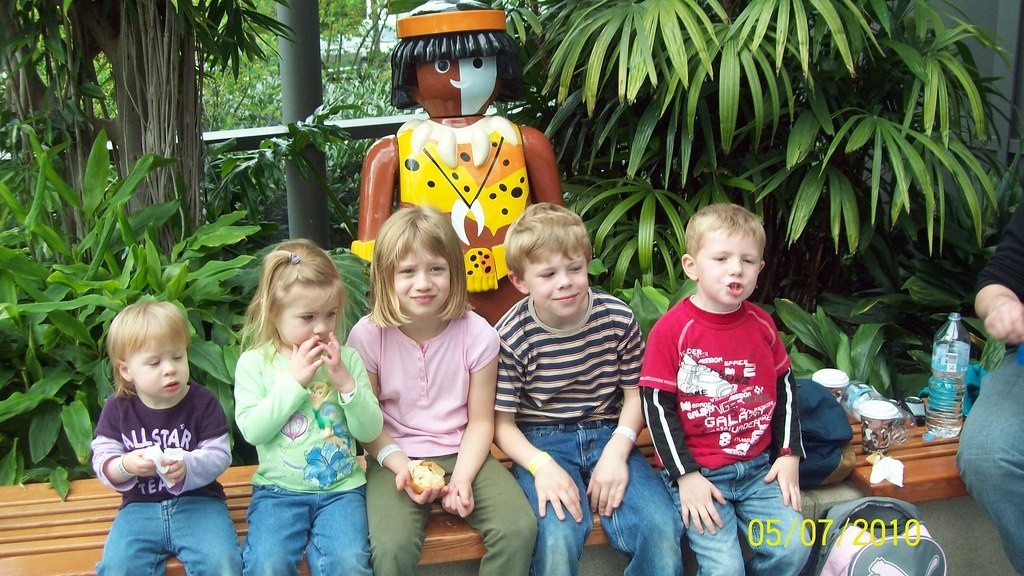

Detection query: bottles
xmin=925 ymin=313 xmax=970 ymax=437
xmin=843 ymin=380 xmax=915 ymax=446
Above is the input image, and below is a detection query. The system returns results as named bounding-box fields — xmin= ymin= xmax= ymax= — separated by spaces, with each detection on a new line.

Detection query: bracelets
xmin=341 ymin=387 xmax=356 ymax=403
xmin=118 ymin=452 xmax=136 ymax=478
xmin=377 ymin=444 xmax=402 ymax=467
xmin=528 ymin=452 xmax=552 ymax=475
xmin=612 ymin=425 xmax=637 ymax=444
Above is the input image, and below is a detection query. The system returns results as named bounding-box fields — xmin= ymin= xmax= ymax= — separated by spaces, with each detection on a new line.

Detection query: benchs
xmin=0 ymin=426 xmax=661 ymax=576
xmin=845 ymin=412 xmax=967 ymax=504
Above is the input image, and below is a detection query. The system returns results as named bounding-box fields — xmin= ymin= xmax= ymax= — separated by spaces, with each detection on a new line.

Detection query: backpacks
xmin=796 ymin=497 xmax=947 ymax=576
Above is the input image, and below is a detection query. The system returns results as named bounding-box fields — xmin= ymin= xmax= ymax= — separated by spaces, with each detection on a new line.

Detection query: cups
xmin=813 ymin=368 xmax=848 ymax=401
xmin=859 ymin=400 xmax=899 ymax=455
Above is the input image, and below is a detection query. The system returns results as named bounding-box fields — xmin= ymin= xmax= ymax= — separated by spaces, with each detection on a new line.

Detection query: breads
xmin=408 ymin=459 xmax=445 ymax=494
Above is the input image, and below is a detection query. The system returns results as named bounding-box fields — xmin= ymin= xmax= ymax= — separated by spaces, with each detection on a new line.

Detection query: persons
xmin=234 ymin=239 xmax=383 ymax=576
xmin=494 ymin=203 xmax=686 ymax=576
xmin=344 ymin=207 xmax=538 ymax=576
xmin=638 ymin=203 xmax=812 ymax=576
xmin=351 ymin=10 xmax=564 ymax=327
xmin=91 ymin=302 xmax=243 ymax=576
xmin=955 ymin=226 xmax=1024 ymax=575
